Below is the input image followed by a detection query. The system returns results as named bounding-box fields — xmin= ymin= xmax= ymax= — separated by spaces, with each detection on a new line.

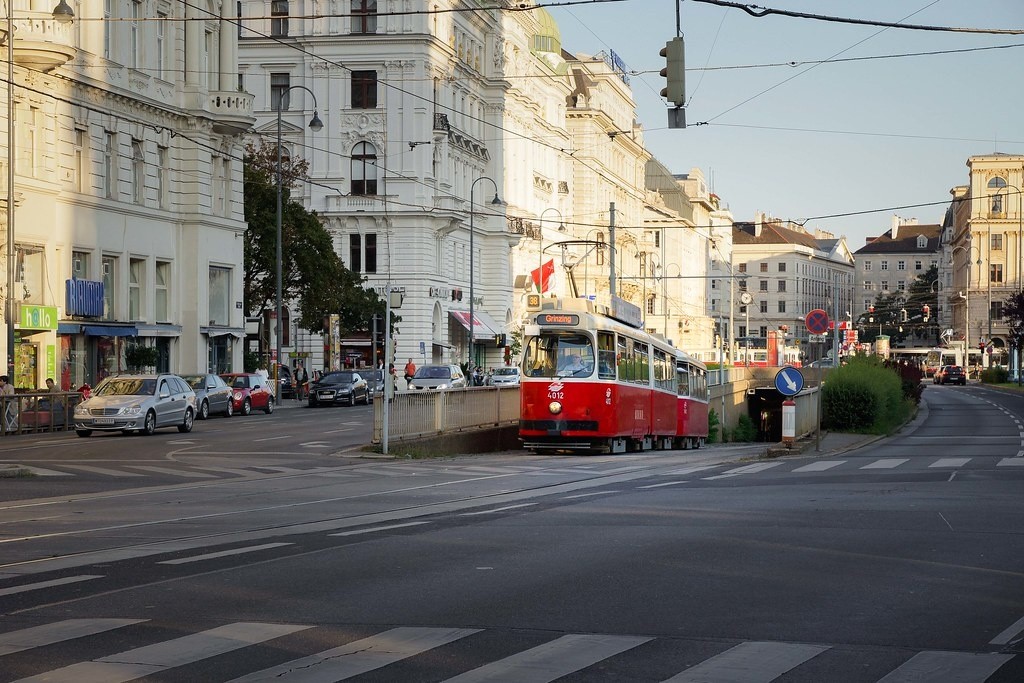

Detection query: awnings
xmin=135 ymin=324 xmax=182 ymax=336
xmin=200 ymin=326 xmax=248 ymax=338
xmin=58 ymin=323 xmax=138 ymax=336
xmin=449 ymin=310 xmax=514 ymax=346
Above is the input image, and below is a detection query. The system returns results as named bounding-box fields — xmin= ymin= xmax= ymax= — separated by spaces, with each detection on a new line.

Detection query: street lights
xmin=539 ymin=207 xmax=566 ymax=305
xmin=468 ymin=175 xmax=503 ymax=389
xmin=275 ymin=85 xmax=324 ymax=408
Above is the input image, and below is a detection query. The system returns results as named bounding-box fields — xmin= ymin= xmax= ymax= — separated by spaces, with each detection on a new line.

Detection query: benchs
xmin=19 ymin=409 xmax=73 ymax=432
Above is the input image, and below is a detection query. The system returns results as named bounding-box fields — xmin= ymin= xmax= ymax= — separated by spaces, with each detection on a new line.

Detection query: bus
xmin=518 ymin=295 xmax=710 ymax=455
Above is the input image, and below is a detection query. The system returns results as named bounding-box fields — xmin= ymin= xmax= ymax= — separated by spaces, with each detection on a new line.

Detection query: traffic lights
xmin=659 ymin=45 xmax=669 ymax=99
xmin=901 ymin=310 xmax=906 ymax=323
xmin=922 ymin=306 xmax=929 ymax=319
xmin=981 ymin=343 xmax=984 ymax=353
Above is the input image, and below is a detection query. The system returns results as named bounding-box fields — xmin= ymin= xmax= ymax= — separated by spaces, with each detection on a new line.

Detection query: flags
xmin=533 ymin=259 xmax=555 ymax=297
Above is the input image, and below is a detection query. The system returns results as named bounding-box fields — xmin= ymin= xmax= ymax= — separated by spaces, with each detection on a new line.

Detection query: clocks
xmin=741 ymin=293 xmax=752 ymax=304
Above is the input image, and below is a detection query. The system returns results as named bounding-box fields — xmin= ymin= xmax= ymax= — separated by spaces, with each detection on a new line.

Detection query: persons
xmin=146 ymin=382 xmax=156 ymax=394
xmin=41 ymin=378 xmax=65 ymax=406
xmin=255 ymin=362 xmax=269 ymax=383
xmin=291 ymin=370 xmax=297 ymax=399
xmin=974 ymin=362 xmax=979 ymax=380
xmin=0 ymin=375 xmax=15 ymax=422
xmin=295 ymin=363 xmax=308 ymax=401
xmin=564 ymin=354 xmax=587 ymax=371
xmin=404 ymin=358 xmax=416 ymax=384
xmin=394 ymin=368 xmax=398 ymax=391
xmin=377 ymin=359 xmax=385 ymax=369
xmin=461 ymin=362 xmax=494 ymax=385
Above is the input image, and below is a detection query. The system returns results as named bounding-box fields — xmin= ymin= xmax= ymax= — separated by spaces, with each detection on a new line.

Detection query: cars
xmin=70 ymin=363 xmax=521 ymax=437
xmin=933 ymin=365 xmax=967 ymax=386
